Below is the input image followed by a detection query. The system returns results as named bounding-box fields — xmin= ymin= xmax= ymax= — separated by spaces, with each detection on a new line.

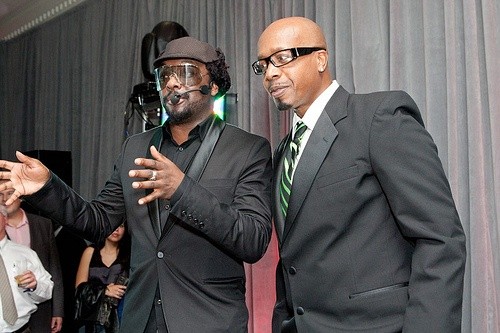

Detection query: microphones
xmin=171 ymin=80 xmax=214 ymax=106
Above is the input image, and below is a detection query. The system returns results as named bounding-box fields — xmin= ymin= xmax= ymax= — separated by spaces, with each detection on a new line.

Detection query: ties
xmin=0 ymin=254 xmax=19 ymax=326
xmin=278 ymin=119 xmax=308 ymax=223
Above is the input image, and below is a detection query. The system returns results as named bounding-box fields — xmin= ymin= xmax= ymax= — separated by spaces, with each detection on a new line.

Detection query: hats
xmin=152 ymin=36 xmax=219 ymax=63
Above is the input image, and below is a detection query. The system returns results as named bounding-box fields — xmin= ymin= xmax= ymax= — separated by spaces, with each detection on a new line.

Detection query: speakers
xmin=21 ymin=150 xmax=73 ymax=232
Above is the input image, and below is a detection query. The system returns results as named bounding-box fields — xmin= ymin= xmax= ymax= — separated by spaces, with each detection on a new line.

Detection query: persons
xmin=74 ymin=219 xmax=129 ymax=333
xmin=257 ymin=16 xmax=467 ymax=333
xmin=0 ymin=205 xmax=54 ymax=333
xmin=0 ymin=179 xmax=65 ymax=333
xmin=0 ymin=36 xmax=273 ymax=333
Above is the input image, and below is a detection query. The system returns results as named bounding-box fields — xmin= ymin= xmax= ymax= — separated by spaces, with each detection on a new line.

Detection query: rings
xmin=149 ymin=171 xmax=157 ymax=180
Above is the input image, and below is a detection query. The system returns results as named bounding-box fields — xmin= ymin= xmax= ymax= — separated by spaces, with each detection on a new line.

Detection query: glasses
xmin=153 ymin=63 xmax=210 ymax=90
xmin=252 ymin=46 xmax=326 ymax=75
